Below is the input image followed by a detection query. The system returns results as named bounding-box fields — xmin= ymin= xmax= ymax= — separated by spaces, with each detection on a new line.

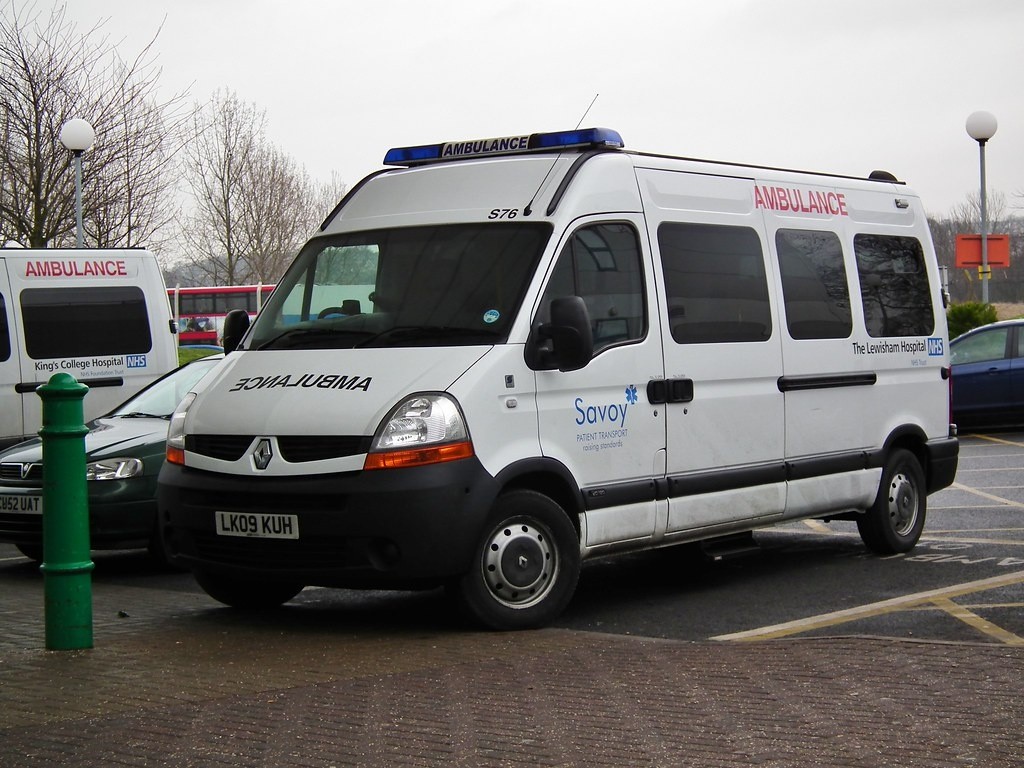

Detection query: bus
xmin=166 ymin=283 xmax=278 ymax=348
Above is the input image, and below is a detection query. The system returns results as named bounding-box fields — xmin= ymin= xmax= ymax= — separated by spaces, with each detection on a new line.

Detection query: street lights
xmin=966 ymin=111 xmax=997 ymax=303
xmin=60 ymin=119 xmax=95 ymax=248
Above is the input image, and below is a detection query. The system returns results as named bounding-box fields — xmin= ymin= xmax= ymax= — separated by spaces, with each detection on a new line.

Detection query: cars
xmin=950 ymin=319 xmax=1024 ymax=432
xmin=0 ymin=351 xmax=228 ymax=580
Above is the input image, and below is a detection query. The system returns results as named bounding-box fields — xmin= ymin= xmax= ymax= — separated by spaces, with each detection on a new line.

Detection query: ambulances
xmin=154 ymin=127 xmax=960 ymax=631
xmin=0 ymin=249 xmax=181 ymax=452
